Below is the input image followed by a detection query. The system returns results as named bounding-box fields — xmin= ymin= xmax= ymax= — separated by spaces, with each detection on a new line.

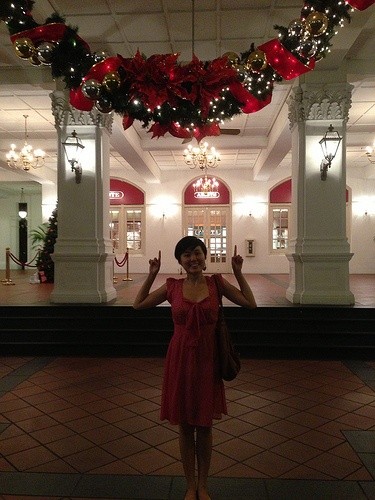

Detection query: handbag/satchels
xmin=213 ymin=273 xmax=242 ymax=382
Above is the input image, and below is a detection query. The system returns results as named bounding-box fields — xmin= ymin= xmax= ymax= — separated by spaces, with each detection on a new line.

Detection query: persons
xmin=133 ymin=236 xmax=257 ymax=500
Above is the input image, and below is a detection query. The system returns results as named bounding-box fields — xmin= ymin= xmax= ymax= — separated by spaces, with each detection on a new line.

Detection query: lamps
xmin=193 ymin=173 xmax=219 ymax=198
xmin=182 ymin=139 xmax=221 ymax=172
xmin=62 ymin=129 xmax=86 ymax=185
xmin=5 ymin=114 xmax=46 ymax=173
xmin=365 ymin=138 xmax=375 ymax=166
xmin=18 ymin=188 xmax=28 ymax=219
xmin=319 ymin=123 xmax=343 ymax=182
